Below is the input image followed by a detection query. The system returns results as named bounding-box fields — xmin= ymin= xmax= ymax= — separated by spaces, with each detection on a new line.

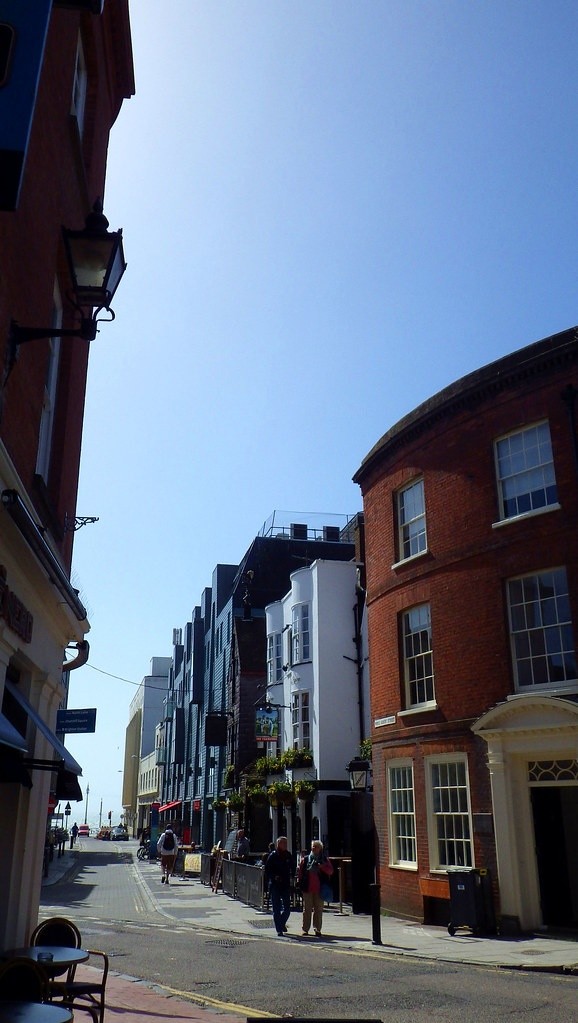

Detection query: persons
xmin=157 ymin=824 xmax=178 ymax=884
xmin=266 ymin=837 xmax=296 ymax=936
xmin=237 ymin=830 xmax=250 ymax=863
xmin=298 ymin=840 xmax=334 ymax=937
xmin=70 ymin=822 xmax=78 ymax=844
xmin=139 ymin=828 xmax=148 ymax=860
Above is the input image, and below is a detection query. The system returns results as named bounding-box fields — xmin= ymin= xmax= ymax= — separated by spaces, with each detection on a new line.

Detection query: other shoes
xmin=277 ymin=925 xmax=288 ymax=936
xmin=302 ymin=931 xmax=308 ymax=935
xmin=314 ymin=929 xmax=321 ymax=936
xmin=161 ymin=876 xmax=169 ymax=884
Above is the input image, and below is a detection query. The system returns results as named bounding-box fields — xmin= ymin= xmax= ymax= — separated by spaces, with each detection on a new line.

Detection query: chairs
xmin=0 ymin=954 xmax=72 ymax=1023
xmin=30 ymin=917 xmax=108 ymax=1023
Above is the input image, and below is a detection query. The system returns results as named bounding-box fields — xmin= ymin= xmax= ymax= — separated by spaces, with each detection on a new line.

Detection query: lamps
xmin=5 ymin=192 xmax=129 ymax=369
xmin=344 ymin=755 xmax=374 ymax=792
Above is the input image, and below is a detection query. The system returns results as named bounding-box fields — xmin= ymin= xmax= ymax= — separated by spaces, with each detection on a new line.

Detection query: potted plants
xmin=211 ymin=799 xmax=227 ymax=812
xmin=246 ymin=784 xmax=266 ymax=808
xmin=274 ymin=781 xmax=290 ymax=800
xmin=293 ymin=781 xmax=315 ymax=799
xmin=222 ymin=766 xmax=235 ymax=788
xmin=227 ymin=793 xmax=245 ymax=812
xmin=254 ymin=747 xmax=312 ymax=775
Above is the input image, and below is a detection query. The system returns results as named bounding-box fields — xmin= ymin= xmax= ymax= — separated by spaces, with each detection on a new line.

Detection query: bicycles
xmin=137 ymin=839 xmax=151 ymax=862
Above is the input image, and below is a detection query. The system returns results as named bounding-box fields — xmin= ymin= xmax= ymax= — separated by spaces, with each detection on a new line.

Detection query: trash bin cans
xmin=447 ymin=868 xmax=498 ymax=939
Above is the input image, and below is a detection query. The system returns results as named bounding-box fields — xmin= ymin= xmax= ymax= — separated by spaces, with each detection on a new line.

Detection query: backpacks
xmin=163 ymin=832 xmax=175 ymax=851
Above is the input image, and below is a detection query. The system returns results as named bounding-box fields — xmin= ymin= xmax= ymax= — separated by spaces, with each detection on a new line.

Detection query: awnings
xmin=158 ymin=801 xmax=181 ymax=813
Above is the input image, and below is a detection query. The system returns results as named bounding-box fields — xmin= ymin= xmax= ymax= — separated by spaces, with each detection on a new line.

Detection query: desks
xmin=6 ymin=945 xmax=98 ymax=1022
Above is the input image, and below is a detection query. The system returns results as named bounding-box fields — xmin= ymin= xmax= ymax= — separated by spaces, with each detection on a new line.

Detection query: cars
xmin=97 ymin=830 xmax=111 ymax=841
xmin=109 ymin=827 xmax=129 ymax=841
xmin=77 ymin=825 xmax=90 ymax=838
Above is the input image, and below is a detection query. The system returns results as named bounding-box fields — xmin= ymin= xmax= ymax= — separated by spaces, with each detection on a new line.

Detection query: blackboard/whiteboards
xmin=171 ymin=848 xmax=192 ymax=877
xmin=184 ymin=854 xmax=201 ymax=872
